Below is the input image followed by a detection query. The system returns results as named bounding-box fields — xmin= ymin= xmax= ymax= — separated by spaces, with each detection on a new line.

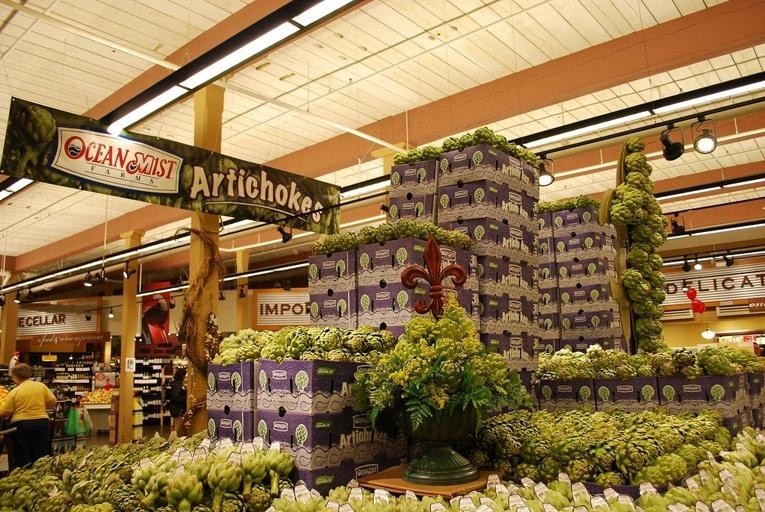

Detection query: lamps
xmin=658 ymin=113 xmax=720 ymax=161
xmin=0 ymin=261 xmax=137 ymax=321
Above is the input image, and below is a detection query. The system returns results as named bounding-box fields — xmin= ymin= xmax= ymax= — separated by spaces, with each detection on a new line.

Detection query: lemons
xmin=84 ymin=388 xmax=118 ymax=405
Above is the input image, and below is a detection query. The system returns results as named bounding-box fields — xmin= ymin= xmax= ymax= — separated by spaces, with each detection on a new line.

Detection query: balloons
xmin=687 ymin=288 xmax=704 ymax=314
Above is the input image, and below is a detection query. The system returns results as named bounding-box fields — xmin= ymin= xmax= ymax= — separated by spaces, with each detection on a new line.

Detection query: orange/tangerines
xmin=0 ymin=385 xmax=10 ymax=407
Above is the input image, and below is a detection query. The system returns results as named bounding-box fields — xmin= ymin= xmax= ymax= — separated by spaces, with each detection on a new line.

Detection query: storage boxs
xmin=204 ymin=353 xmax=410 ymax=497
xmin=537 ymin=344 xmax=764 ymax=434
xmin=298 ymin=235 xmax=481 ymax=349
xmin=388 ymin=147 xmax=535 ymax=387
xmin=532 ymin=198 xmax=637 ymax=353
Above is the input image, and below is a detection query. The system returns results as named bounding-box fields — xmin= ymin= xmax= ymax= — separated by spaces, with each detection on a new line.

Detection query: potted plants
xmin=357 ymin=289 xmax=528 ymax=484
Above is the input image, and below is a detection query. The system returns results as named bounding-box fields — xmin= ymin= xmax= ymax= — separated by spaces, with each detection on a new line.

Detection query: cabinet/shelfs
xmin=18 ymin=342 xmax=199 ymax=438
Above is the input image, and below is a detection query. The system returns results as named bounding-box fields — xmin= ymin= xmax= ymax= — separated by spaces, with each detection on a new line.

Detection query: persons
xmin=166 ymin=369 xmax=188 ymax=434
xmin=0 ymin=362 xmax=57 ymax=469
